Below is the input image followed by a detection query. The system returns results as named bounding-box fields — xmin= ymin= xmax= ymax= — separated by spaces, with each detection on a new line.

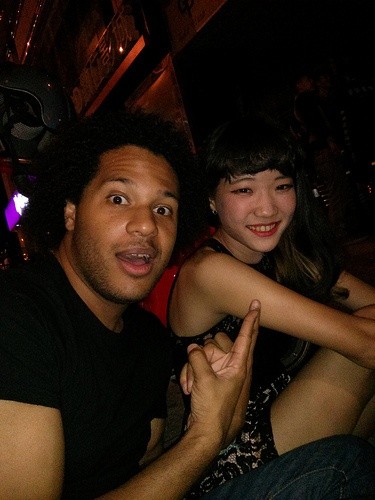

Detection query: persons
xmin=166 ymin=118 xmax=375 ymax=500
xmin=0 ymin=113 xmax=375 ymax=500
xmin=294 ymin=73 xmax=369 ymax=247
